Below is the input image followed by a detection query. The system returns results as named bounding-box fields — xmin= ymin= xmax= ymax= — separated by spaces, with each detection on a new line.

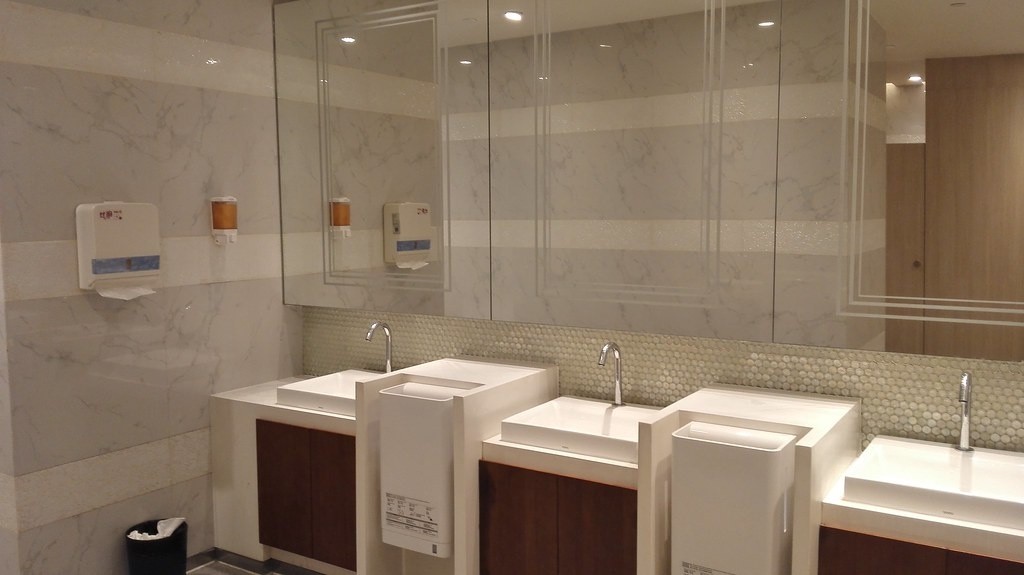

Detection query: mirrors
xmin=271 ymin=0 xmax=1024 ymax=362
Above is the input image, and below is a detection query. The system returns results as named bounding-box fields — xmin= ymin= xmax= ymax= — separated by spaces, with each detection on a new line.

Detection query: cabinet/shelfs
xmin=817 ymin=524 xmax=1024 ymax=575
xmin=478 ymin=459 xmax=638 ymax=575
xmin=255 ymin=418 xmax=358 ymax=572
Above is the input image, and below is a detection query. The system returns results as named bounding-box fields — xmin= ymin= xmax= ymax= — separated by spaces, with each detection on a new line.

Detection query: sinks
xmin=501 ymin=395 xmax=665 ymax=463
xmin=842 ymin=434 xmax=1024 ymax=531
xmin=276 ymin=368 xmax=386 ymax=417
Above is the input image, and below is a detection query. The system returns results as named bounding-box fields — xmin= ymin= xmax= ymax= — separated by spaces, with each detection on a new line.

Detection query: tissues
xmin=76 ymin=200 xmax=162 ymax=301
xmin=383 ymin=201 xmax=433 ymax=271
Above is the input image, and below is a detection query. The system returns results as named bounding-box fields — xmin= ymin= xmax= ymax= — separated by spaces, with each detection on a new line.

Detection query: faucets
xmin=365 ymin=321 xmax=392 ymax=373
xmin=958 ymin=372 xmax=973 ymax=452
xmin=598 ymin=342 xmax=622 ymax=405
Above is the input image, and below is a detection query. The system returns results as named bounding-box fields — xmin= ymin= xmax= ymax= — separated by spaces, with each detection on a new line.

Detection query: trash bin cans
xmin=124 ymin=517 xmax=187 ymax=575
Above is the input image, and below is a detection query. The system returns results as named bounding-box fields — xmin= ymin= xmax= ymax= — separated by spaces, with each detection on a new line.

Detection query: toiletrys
xmin=328 ymin=197 xmax=352 ymax=242
xmin=209 ymin=196 xmax=239 ymax=247
